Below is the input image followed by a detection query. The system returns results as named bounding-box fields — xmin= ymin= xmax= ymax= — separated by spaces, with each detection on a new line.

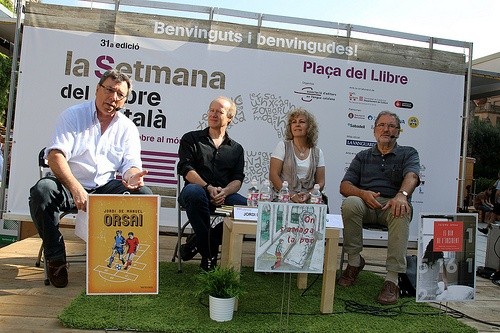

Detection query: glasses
xmin=100 ymin=85 xmax=127 ymax=99
xmin=376 ymin=123 xmax=399 ymax=130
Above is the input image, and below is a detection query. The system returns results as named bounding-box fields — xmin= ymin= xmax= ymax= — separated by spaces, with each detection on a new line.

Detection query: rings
xmin=401 ymin=205 xmax=406 ymax=206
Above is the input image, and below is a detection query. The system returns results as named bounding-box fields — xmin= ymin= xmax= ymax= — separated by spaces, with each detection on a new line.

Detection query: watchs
xmin=398 ymin=190 xmax=409 ymax=198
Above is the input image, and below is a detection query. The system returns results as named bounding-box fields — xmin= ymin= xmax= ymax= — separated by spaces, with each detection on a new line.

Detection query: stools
xmin=338 ymin=225 xmax=392 ymax=279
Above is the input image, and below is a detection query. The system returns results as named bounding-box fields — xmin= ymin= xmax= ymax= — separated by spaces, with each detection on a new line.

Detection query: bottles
xmin=309 ymin=183 xmax=323 ymax=204
xmin=279 ymin=180 xmax=290 ymax=204
xmin=246 ymin=179 xmax=259 ymax=206
xmin=260 ymin=179 xmax=273 ymax=202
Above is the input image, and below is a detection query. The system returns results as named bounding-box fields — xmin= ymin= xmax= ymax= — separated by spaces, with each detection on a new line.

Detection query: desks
xmin=220 ymin=217 xmax=340 ymax=314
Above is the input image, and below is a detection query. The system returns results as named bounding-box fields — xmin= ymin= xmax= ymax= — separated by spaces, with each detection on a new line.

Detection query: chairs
xmin=171 ymin=159 xmax=223 ymax=272
xmin=35 ymin=147 xmax=76 ymax=285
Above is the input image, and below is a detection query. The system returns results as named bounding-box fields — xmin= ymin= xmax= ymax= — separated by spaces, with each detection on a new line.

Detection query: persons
xmin=264 ymin=109 xmax=325 ymax=277
xmin=475 ymin=173 xmax=500 ymax=235
xmin=338 ymin=111 xmax=421 ymax=306
xmin=176 ymin=95 xmax=247 ymax=273
xmin=419 ymin=239 xmax=447 ymax=300
xmin=29 ymin=70 xmax=156 ymax=289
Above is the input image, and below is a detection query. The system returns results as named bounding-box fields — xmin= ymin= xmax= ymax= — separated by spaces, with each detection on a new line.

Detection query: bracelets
xmin=291 ymin=193 xmax=296 ymax=199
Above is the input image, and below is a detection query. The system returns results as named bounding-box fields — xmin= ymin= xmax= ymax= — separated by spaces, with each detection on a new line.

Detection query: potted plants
xmin=193 ymin=263 xmax=243 ymax=322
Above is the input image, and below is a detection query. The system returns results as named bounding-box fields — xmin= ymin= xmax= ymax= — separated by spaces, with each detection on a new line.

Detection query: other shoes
xmin=376 ymin=280 xmax=399 ymax=305
xmin=200 ymin=255 xmax=217 ymax=273
xmin=49 ymin=259 xmax=68 ymax=288
xmin=337 ymin=255 xmax=365 ymax=287
xmin=478 ymin=226 xmax=489 ymax=234
xmin=180 ymin=233 xmax=198 ymax=261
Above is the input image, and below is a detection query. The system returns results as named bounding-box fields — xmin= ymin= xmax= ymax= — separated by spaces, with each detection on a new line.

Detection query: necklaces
xmin=293 ymin=141 xmax=307 ymax=157
